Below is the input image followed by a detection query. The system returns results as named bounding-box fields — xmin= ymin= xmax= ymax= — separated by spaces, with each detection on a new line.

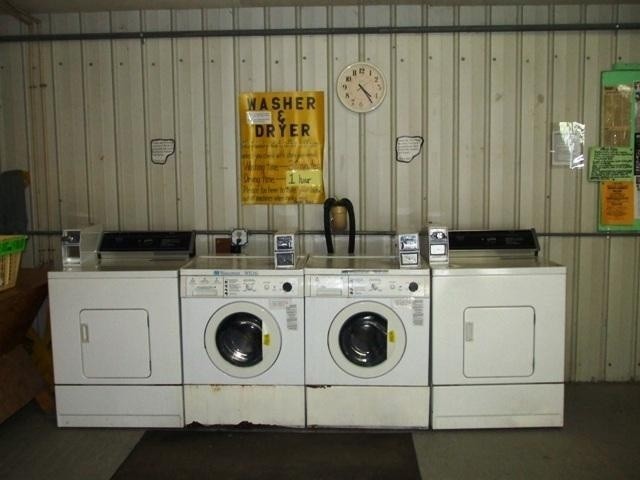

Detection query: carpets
xmin=107 ymin=422 xmax=421 ymax=479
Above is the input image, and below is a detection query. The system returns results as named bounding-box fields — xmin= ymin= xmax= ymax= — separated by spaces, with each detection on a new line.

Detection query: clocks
xmin=335 ymin=60 xmax=389 ymax=115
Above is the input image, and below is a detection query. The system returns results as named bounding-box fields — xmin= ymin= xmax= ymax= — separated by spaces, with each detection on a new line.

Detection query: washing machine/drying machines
xmin=304 ymin=256 xmax=429 ymax=434
xmin=48 ymin=231 xmax=194 ymax=428
xmin=430 ymin=227 xmax=568 ymax=431
xmin=179 ymin=255 xmax=306 ymax=431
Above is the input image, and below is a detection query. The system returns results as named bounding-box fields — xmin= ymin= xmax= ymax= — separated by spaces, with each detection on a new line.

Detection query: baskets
xmin=0 ymin=234 xmax=29 ymax=292
